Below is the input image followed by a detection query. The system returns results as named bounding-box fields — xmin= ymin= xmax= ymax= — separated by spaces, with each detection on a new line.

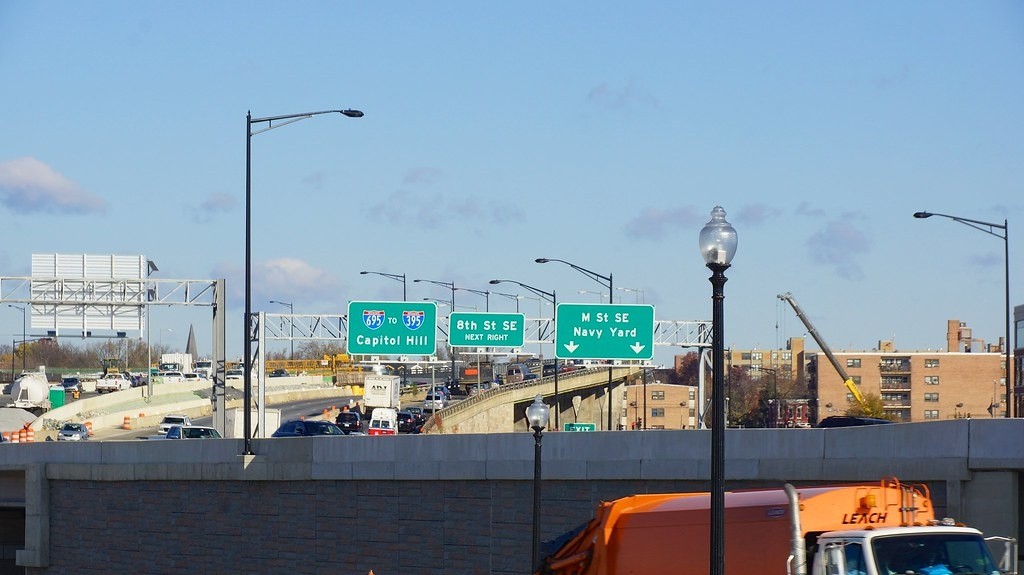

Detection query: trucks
xmin=533 ymin=474 xmax=1019 ymax=575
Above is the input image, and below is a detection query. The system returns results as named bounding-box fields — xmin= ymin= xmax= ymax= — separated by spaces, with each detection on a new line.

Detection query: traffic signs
xmin=554 ymin=302 xmax=655 ymax=360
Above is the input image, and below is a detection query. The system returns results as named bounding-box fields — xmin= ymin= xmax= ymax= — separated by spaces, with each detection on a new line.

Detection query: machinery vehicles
xmin=776 ymin=291 xmax=876 ymax=419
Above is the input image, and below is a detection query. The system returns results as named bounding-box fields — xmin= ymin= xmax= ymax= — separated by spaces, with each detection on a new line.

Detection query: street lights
xmin=360 ymin=271 xmax=407 ymax=388
xmin=525 ymin=393 xmax=552 ymax=575
xmin=697 ymin=204 xmax=739 ymax=575
xmin=242 ymin=106 xmax=365 ymax=455
xmin=488 ymin=279 xmax=559 ymax=431
xmin=268 ymin=300 xmax=295 ymax=363
xmin=412 ymin=279 xmax=455 ymax=380
xmin=7 ymin=304 xmax=26 ymax=372
xmin=577 ymin=286 xmax=645 ymax=376
xmin=423 ymin=297 xmax=481 ymax=399
xmin=535 ymin=258 xmax=613 ymax=431
xmin=490 ymin=291 xmax=520 ymax=365
xmin=520 ymin=300 xmax=544 ymax=384
xmin=912 ymin=211 xmax=1012 ymax=418
xmin=452 ymin=287 xmax=489 ymax=364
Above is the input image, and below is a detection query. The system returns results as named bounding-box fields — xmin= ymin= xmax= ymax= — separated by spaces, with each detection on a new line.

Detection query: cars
xmin=54 ymin=352 xmax=592 ymax=446
